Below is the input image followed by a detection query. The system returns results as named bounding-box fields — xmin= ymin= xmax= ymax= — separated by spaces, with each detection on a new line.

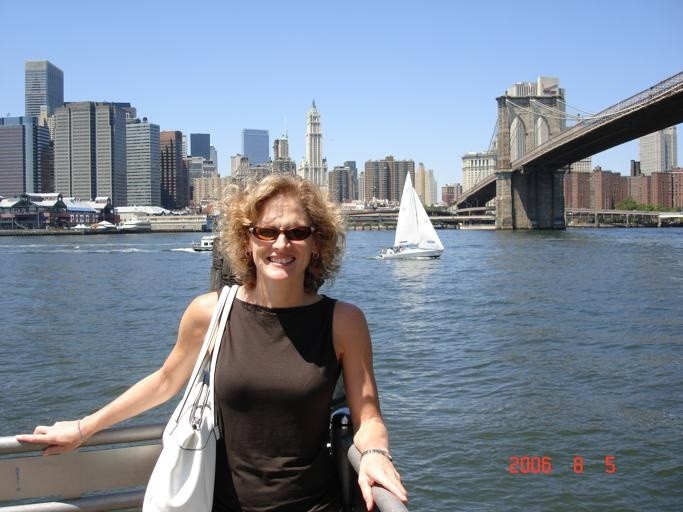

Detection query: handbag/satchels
xmin=143 ymin=284 xmax=240 ymax=510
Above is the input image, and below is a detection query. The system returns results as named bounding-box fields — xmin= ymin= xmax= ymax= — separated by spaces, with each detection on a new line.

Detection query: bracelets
xmin=359 ymin=446 xmax=391 ymax=462
xmin=76 ymin=417 xmax=86 ymax=443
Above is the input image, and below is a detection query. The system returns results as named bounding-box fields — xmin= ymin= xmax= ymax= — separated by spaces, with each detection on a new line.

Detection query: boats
xmin=191 ymin=235 xmax=218 ymax=252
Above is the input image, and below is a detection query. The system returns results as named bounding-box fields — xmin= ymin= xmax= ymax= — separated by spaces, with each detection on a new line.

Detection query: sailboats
xmin=379 ymin=171 xmax=444 ymax=261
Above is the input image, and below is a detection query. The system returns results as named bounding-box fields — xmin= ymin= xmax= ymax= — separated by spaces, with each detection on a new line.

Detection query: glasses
xmin=248 ymin=226 xmax=315 ymax=241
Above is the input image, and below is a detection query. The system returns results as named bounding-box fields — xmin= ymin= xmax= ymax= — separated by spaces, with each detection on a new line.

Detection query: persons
xmin=15 ymin=167 xmax=408 ymax=512
